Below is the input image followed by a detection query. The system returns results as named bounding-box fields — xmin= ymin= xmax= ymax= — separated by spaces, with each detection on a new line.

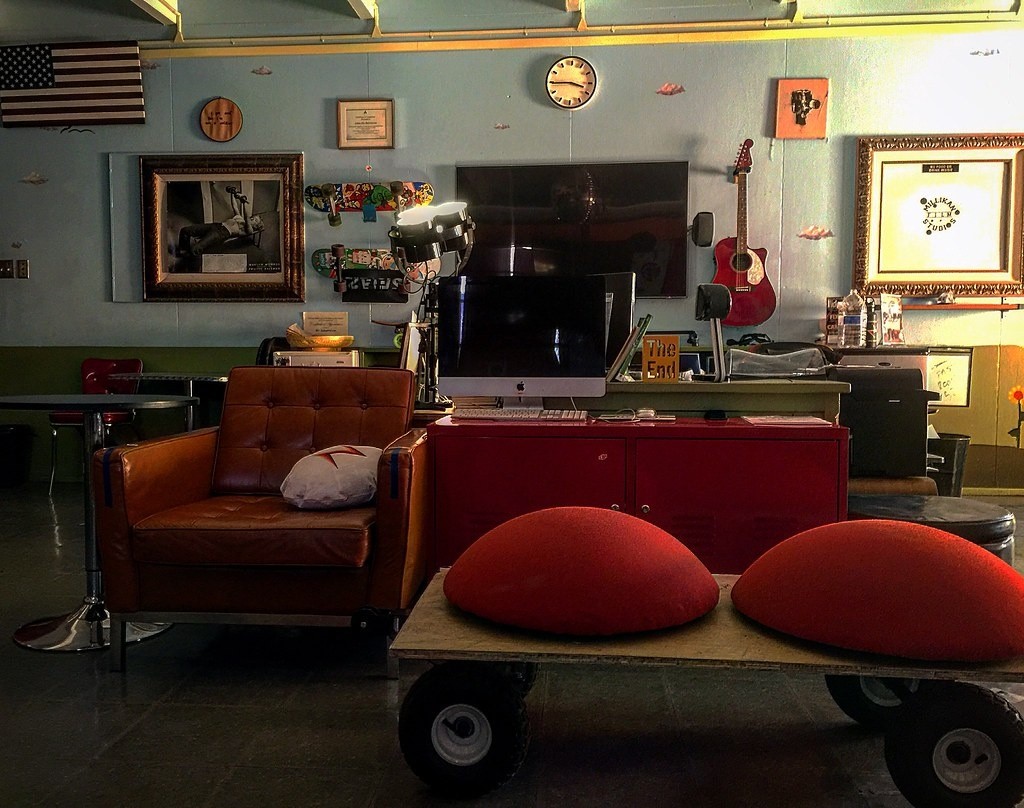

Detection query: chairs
xmin=46 ymin=359 xmax=144 ymax=500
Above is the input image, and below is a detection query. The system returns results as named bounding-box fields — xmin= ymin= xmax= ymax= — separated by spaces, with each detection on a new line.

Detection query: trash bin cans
xmin=926 ymin=432 xmax=972 ymax=498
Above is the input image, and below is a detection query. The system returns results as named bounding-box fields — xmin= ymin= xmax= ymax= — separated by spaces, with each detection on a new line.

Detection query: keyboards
xmin=450 ymin=409 xmax=588 ymax=421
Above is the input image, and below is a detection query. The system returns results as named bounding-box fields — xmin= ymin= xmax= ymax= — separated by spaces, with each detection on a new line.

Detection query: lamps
xmin=386 ymin=201 xmax=473 ymax=411
xmin=690 ymin=282 xmax=733 ymax=383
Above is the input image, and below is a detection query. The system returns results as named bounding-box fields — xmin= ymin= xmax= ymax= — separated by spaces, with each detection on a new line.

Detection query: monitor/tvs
xmin=437 ymin=275 xmax=606 ymax=409
xmin=592 ymin=272 xmax=635 ymax=368
xmin=454 ymin=160 xmax=690 ymax=299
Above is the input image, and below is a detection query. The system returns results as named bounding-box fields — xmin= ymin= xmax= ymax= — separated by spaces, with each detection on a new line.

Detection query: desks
xmin=833 ymin=493 xmax=1018 ymax=568
xmin=0 ymin=393 xmax=203 ymax=654
xmin=111 ymin=374 xmax=229 ymax=433
xmin=542 ymin=365 xmax=853 ymax=419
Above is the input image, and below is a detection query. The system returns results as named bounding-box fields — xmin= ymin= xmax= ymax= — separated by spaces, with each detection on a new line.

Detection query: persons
xmin=168 ymin=216 xmax=265 ymax=272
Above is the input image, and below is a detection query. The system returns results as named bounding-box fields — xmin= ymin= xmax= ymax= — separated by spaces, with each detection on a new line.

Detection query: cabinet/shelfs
xmin=422 ymin=409 xmax=851 ymax=575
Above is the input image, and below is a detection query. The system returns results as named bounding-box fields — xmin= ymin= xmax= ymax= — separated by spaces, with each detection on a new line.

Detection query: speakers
xmin=693 ymin=212 xmax=714 ymax=247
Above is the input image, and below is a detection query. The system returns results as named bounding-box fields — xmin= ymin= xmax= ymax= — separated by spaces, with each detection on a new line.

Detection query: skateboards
xmin=311 ymin=244 xmax=443 ymax=292
xmin=305 ymin=181 xmax=436 ymax=226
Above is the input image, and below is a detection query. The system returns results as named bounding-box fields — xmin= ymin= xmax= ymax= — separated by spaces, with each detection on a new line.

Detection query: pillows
xmin=279 ymin=441 xmax=382 ymax=513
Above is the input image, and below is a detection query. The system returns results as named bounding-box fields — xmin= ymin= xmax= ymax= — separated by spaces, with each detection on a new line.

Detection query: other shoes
xmin=168 ymin=244 xmax=182 ymax=257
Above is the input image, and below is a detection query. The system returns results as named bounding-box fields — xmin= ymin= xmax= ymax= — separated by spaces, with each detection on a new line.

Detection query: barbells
xmin=225 ymin=185 xmax=249 ymax=204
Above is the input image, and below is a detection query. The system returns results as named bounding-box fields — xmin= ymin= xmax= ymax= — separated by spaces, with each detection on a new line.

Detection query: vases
xmin=137 ymin=152 xmax=306 ymax=304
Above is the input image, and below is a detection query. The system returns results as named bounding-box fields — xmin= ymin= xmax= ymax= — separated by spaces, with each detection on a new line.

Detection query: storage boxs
xmin=274 ymin=349 xmax=360 ymax=367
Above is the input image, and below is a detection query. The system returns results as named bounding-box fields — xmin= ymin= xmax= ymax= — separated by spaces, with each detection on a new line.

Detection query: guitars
xmin=710 ymin=138 xmax=780 ymax=328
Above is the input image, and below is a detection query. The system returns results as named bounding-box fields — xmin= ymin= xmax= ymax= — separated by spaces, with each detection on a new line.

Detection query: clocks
xmin=544 ymin=55 xmax=600 ymax=111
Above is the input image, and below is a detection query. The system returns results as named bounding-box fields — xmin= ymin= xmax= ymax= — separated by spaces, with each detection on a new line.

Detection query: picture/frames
xmin=927 ymin=345 xmax=974 ymax=406
xmin=399 ymin=322 xmax=432 ymax=370
xmin=856 ymin=135 xmax=1024 ymax=301
xmin=334 ymin=97 xmax=398 ymax=150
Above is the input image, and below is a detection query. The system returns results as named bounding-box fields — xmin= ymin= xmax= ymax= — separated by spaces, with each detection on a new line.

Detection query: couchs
xmin=92 ymin=364 xmax=426 ymax=677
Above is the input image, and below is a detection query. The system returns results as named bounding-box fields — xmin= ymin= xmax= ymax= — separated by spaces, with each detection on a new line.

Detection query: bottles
xmin=866 ymin=297 xmax=877 ymax=347
xmin=842 ymin=288 xmax=866 ymax=347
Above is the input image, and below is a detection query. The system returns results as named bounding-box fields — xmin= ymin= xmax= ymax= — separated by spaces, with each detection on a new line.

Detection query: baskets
xmin=286 ymin=322 xmax=354 ymax=347
